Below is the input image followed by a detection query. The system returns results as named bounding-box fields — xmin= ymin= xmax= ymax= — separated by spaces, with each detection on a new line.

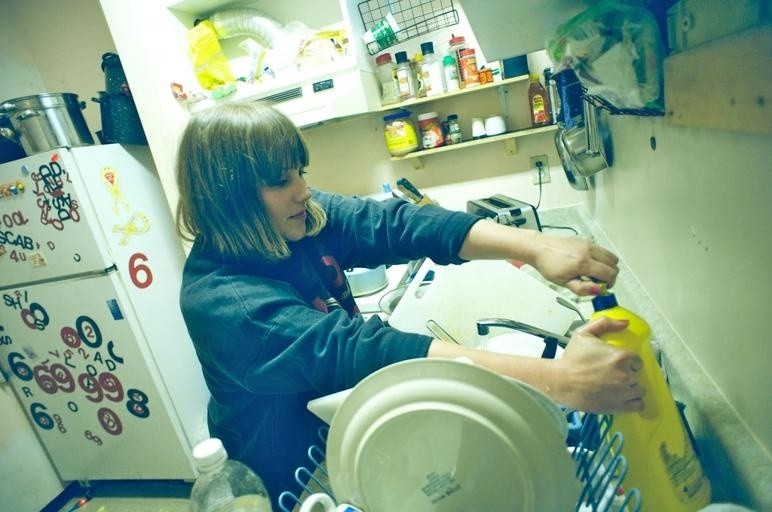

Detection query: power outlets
xmin=528 ymin=155 xmax=551 ymax=186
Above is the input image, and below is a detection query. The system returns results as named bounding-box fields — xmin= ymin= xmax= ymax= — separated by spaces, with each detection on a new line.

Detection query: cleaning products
xmin=587 ymin=281 xmax=713 ymax=512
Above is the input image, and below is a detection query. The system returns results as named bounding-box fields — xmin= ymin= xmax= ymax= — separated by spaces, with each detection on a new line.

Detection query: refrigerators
xmin=0 ymin=144 xmax=211 ymax=484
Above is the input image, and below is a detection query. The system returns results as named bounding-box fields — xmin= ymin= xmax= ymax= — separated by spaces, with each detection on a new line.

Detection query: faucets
xmin=477 ymin=296 xmax=587 ymax=349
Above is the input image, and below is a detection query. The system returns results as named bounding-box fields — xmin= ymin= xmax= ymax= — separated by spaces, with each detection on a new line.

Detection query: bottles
xmin=450 ymin=36 xmax=467 ymax=89
xmin=439 ymin=118 xmax=453 ymax=145
xmin=383 ymin=111 xmax=421 ymax=156
xmin=408 ymin=56 xmax=427 ymax=97
xmin=375 ymin=53 xmax=404 ymax=105
xmin=414 ymin=51 xmax=434 ymax=98
xmin=420 ymin=41 xmax=448 ymax=97
xmin=478 ymin=67 xmax=494 ymax=85
xmin=188 ymin=437 xmax=272 ymax=512
xmin=417 ymin=111 xmax=445 ymax=149
xmin=392 ymin=50 xmax=419 ymax=101
xmin=442 ymin=55 xmax=460 ymax=92
xmin=447 ymin=114 xmax=463 ymax=144
xmin=528 ymin=72 xmax=551 ymax=128
xmin=461 ymin=48 xmax=482 ymax=88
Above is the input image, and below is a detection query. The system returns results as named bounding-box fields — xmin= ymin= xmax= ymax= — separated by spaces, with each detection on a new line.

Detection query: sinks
xmin=470 ymin=332 xmax=565 ymax=361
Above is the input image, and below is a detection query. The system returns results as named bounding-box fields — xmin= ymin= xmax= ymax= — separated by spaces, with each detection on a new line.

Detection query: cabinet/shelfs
xmin=378 ymin=73 xmax=566 ymax=169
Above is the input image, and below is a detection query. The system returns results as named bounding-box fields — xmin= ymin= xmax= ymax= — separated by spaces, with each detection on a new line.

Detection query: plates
xmin=326 ymin=358 xmax=579 ymax=512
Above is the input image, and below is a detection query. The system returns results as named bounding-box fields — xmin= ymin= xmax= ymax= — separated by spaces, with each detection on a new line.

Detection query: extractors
xmin=189 ymin=57 xmax=368 ymax=132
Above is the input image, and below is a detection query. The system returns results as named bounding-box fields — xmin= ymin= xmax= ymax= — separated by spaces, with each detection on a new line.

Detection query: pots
xmin=554 ymin=126 xmax=591 ymax=192
xmin=562 ymin=123 xmax=610 ymax=179
xmin=1 ymin=93 xmax=94 ymax=159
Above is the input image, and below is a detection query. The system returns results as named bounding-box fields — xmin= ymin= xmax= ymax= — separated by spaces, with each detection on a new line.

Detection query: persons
xmin=177 ymin=98 xmax=647 ymax=512
